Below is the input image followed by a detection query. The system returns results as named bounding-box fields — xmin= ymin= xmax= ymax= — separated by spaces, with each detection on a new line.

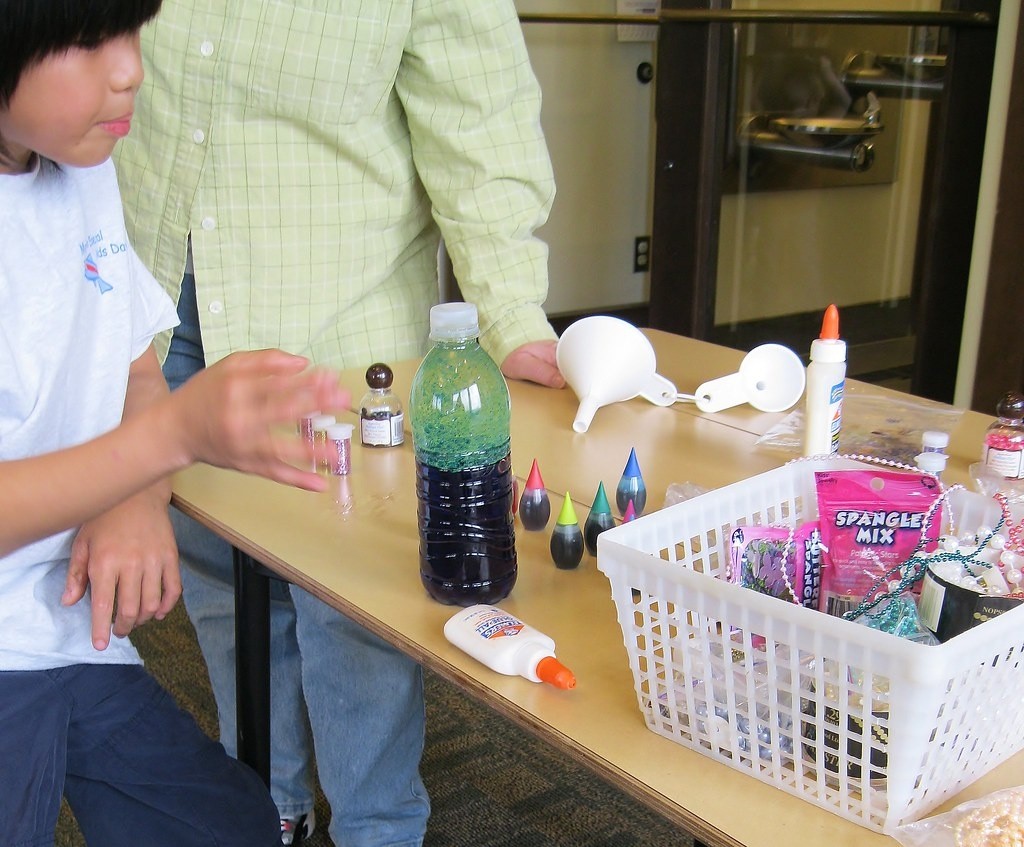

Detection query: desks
xmin=169 ymin=328 xmax=1024 ymax=847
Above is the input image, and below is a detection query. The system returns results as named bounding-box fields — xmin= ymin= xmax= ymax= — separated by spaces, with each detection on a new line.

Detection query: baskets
xmin=596 ymin=454 xmax=1023 ymax=836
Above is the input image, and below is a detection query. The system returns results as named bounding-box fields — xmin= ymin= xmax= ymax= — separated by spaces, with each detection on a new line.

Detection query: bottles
xmin=442 ymin=602 xmax=578 ymax=691
xmin=803 ymin=303 xmax=848 ymax=458
xmin=410 ymin=302 xmax=517 ymax=607
xmin=914 ymin=392 xmax=1024 ymax=487
xmin=358 ymin=362 xmax=401 ymax=446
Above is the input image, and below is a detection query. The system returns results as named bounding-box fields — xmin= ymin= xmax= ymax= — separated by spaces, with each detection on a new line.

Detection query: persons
xmin=0 ymin=1 xmax=352 ymax=847
xmin=113 ymin=0 xmax=567 ymax=847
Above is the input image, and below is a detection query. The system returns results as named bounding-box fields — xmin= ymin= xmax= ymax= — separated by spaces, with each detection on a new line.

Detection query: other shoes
xmin=279 ymin=807 xmax=316 ymax=846
